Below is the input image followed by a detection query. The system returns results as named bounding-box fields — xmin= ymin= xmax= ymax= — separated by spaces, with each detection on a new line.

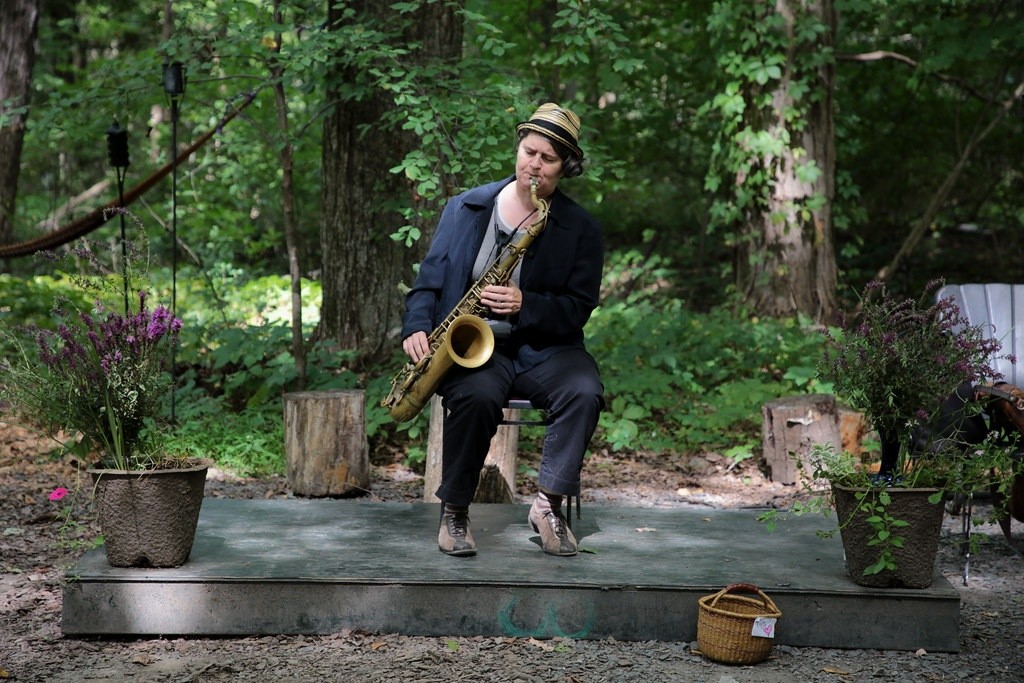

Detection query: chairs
xmin=943 ymin=381 xmax=1024 ymax=544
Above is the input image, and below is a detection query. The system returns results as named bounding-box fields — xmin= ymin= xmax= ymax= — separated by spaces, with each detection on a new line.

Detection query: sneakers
xmin=528 ymin=498 xmax=577 ymax=555
xmin=438 ymin=514 xmax=477 ymax=553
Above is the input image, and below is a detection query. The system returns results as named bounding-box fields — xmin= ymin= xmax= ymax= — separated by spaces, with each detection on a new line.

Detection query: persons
xmin=399 ymin=102 xmax=605 ymax=556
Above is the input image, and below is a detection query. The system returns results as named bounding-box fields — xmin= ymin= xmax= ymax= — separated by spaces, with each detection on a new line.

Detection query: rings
xmin=502 ymin=302 xmax=504 ymax=309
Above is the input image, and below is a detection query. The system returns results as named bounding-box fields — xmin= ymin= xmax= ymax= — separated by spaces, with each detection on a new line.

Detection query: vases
xmin=827 ymin=473 xmax=949 ymax=593
xmin=87 ymin=454 xmax=218 ymax=569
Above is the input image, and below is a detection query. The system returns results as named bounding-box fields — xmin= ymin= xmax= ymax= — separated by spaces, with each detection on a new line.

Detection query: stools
xmin=435 ymin=393 xmax=581 ymax=538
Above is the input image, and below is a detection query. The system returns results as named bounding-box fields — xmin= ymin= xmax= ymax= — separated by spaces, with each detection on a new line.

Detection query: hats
xmin=515 ymin=102 xmax=584 ymax=159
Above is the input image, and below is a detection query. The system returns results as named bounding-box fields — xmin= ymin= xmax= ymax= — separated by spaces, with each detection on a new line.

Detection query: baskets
xmin=697 ymin=582 xmax=783 ymax=663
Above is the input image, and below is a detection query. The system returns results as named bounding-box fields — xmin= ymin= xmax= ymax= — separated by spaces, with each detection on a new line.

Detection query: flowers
xmin=0 ymin=207 xmax=182 ymax=467
xmin=737 ymin=274 xmax=1024 ymax=578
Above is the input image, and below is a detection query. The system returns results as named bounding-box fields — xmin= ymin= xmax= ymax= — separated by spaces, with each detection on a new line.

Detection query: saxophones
xmin=379 ymin=172 xmax=549 ymax=423
